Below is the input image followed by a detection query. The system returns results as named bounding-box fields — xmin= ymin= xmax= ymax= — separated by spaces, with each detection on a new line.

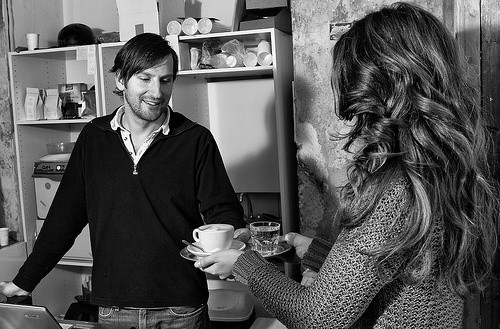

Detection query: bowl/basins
xmin=46 ymin=142 xmax=76 ymax=153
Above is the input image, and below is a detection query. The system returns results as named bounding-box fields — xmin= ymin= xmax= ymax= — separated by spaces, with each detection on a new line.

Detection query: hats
xmin=57 ymin=23 xmax=103 ymax=46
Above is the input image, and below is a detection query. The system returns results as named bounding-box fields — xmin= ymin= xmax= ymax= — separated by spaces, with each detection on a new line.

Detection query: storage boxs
xmin=207 ymin=289 xmax=256 ymax=329
xmin=58 ymin=84 xmax=87 ymax=118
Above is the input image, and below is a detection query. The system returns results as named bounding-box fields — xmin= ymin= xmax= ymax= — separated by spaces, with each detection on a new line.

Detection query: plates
xmin=187 ymin=239 xmax=246 ymax=259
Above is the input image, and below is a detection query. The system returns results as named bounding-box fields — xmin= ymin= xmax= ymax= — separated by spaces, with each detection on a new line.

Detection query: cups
xmin=193 ymin=224 xmax=235 ymax=252
xmin=226 ymin=54 xmax=243 ymax=68
xmin=242 ymin=51 xmax=260 ymax=68
xmin=249 ymin=222 xmax=280 ymax=255
xmin=25 ymin=33 xmax=40 ymax=50
xmin=166 ymin=17 xmax=212 ymax=35
xmin=0 ymin=228 xmax=9 ymax=246
xmin=257 ymin=41 xmax=272 ymax=67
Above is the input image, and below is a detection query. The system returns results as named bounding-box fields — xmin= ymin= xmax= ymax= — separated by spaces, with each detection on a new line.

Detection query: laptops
xmin=0 ymin=303 xmax=100 ymax=329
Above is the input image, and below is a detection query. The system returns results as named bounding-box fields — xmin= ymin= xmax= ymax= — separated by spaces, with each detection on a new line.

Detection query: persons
xmin=0 ymin=32 xmax=245 ymax=329
xmin=194 ymin=2 xmax=500 ymax=329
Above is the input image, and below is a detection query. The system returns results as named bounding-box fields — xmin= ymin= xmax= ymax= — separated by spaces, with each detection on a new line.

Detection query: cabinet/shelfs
xmin=9 ymin=42 xmax=124 ymax=267
xmin=164 ymin=27 xmax=293 ymax=277
